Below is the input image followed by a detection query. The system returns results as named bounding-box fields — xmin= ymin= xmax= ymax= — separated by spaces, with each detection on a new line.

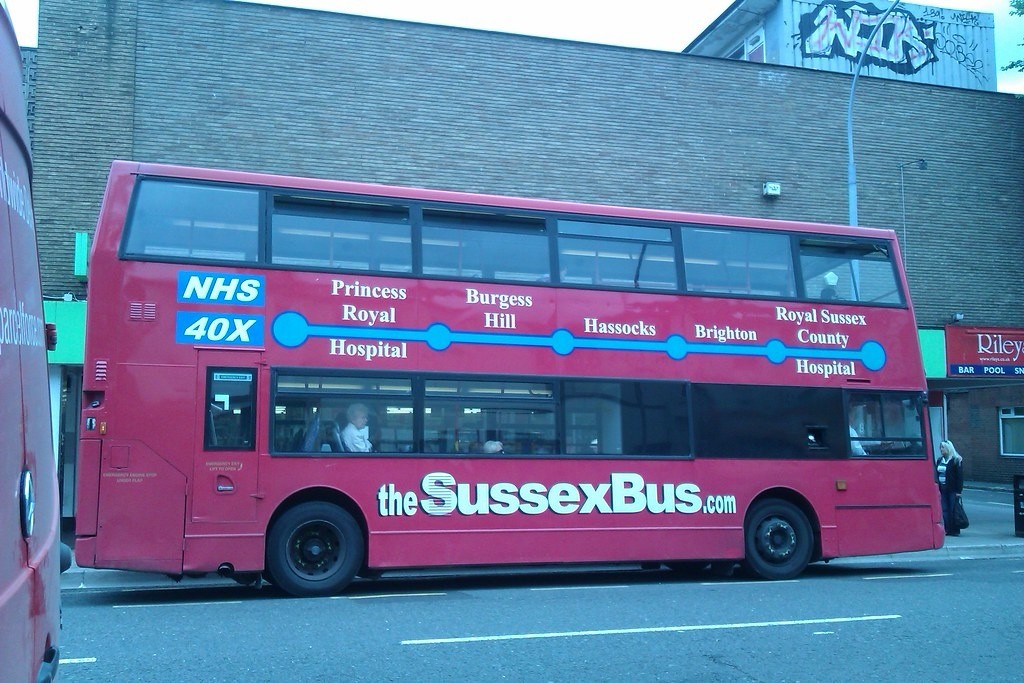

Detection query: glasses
xmin=944 ymin=441 xmax=949 ymax=444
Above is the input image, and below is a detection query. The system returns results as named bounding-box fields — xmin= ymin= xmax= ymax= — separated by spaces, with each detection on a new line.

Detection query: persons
xmin=469 ymin=440 xmax=504 ymax=454
xmin=341 ymin=397 xmax=386 ymax=451
xmin=936 ymin=440 xmax=963 ymax=535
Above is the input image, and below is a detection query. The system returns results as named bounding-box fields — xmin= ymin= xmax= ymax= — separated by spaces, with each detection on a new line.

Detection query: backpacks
xmin=953 ymin=496 xmax=969 ymax=530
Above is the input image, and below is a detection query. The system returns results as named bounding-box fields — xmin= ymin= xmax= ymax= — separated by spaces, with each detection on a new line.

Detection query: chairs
xmin=298 ymin=411 xmax=322 ymax=453
xmin=323 ymin=418 xmax=345 ymax=453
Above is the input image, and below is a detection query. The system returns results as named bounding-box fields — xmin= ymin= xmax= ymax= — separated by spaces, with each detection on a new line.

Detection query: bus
xmin=0 ymin=1 xmax=68 ymax=683
xmin=74 ymin=157 xmax=949 ymax=598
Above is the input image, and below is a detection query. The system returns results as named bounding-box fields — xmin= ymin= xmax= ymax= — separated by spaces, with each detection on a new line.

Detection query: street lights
xmin=899 ymin=155 xmax=928 ymax=272
